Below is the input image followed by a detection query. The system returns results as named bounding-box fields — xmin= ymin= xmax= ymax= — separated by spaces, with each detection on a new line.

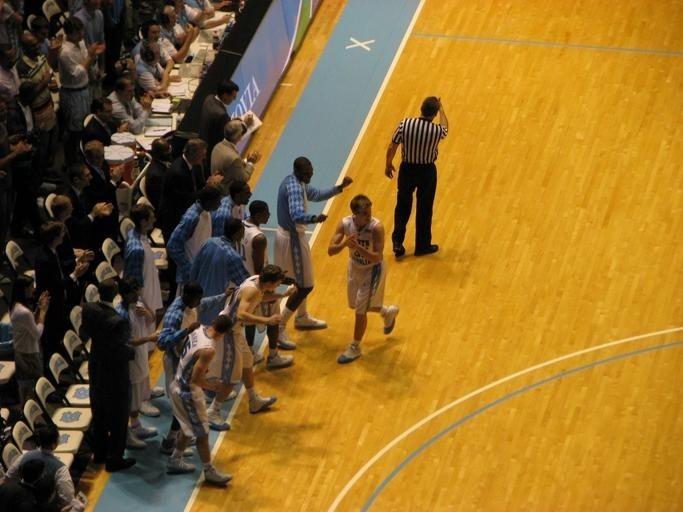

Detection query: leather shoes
xmin=93 ymin=455 xmax=136 ymax=472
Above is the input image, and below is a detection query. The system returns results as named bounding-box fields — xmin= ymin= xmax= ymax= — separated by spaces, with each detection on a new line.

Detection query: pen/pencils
xmin=154 ymin=128 xmax=166 ymax=131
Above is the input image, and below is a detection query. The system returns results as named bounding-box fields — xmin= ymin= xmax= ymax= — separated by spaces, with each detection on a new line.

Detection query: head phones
xmin=119 ymin=57 xmax=131 ymax=76
xmin=143 ymin=39 xmax=155 ymax=62
xmin=158 ymin=4 xmax=168 ymax=23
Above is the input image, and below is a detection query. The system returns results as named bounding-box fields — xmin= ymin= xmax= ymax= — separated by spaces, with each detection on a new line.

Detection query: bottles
xmin=212 ymin=31 xmax=219 ymax=48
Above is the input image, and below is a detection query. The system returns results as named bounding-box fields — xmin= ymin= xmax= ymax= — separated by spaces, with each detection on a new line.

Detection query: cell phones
xmin=186 ymin=56 xmax=193 ymax=63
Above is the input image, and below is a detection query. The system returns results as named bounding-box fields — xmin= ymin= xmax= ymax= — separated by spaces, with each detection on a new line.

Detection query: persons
xmin=0 ymin=1 xmax=297 ymax=511
xmin=383 ymin=97 xmax=450 ymax=259
xmin=327 ymin=194 xmax=400 ymax=365
xmin=273 ymin=154 xmax=354 ymax=352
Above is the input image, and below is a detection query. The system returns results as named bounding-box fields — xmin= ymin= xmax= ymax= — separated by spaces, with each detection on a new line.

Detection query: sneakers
xmin=415 ymin=245 xmax=438 ymax=256
xmin=252 ymin=349 xmax=265 ymax=365
xmin=295 ymin=313 xmax=327 ymax=330
xmin=277 ymin=334 xmax=296 ymax=350
xmin=204 ymin=463 xmax=232 ymax=485
xmin=161 ymin=428 xmax=197 ymax=474
xmin=224 ymin=388 xmax=237 ymax=400
xmin=338 ymin=344 xmax=361 ymax=364
xmin=150 ymin=390 xmax=164 ymax=397
xmin=266 ymin=353 xmax=292 ymax=370
xmin=138 ymin=401 xmax=160 ymax=416
xmin=383 ymin=305 xmax=399 ymax=335
xmin=393 ymin=245 xmax=405 ymax=256
xmin=126 ymin=421 xmax=158 ymax=449
xmin=249 ymin=395 xmax=276 ymax=413
xmin=207 ymin=409 xmax=230 ymax=430
